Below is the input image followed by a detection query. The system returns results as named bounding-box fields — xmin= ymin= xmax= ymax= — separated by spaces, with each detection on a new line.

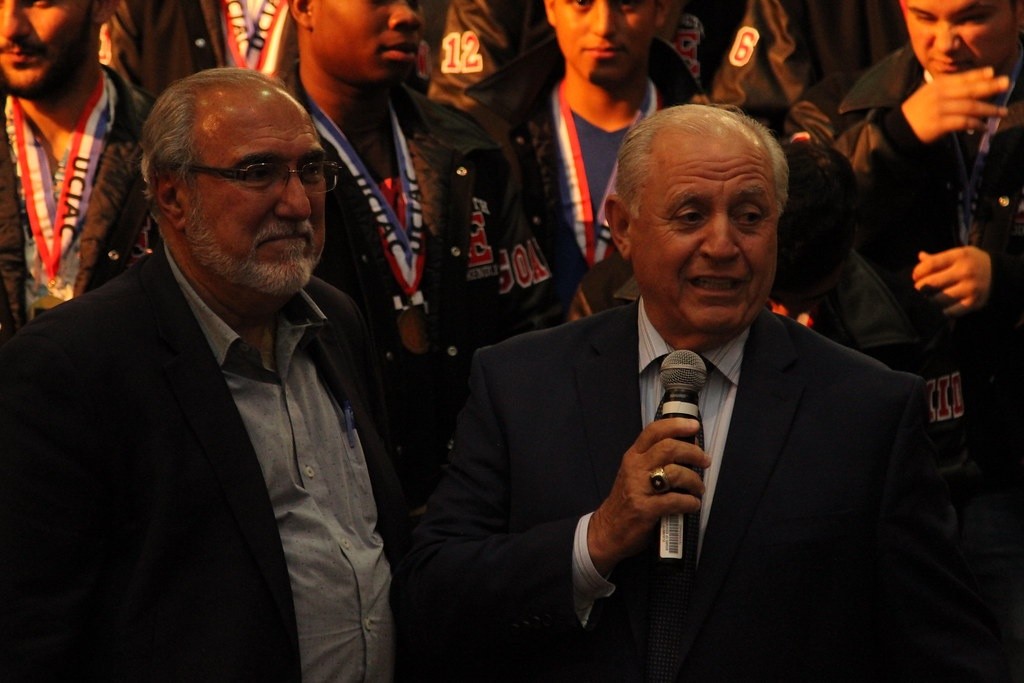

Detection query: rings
xmin=649 ymin=468 xmax=670 ymax=494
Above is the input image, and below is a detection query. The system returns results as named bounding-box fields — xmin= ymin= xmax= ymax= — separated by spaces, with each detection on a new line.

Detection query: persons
xmin=0 ymin=0 xmax=161 ymax=344
xmin=281 ymin=0 xmax=559 ymax=484
xmin=0 ymin=68 xmax=411 ymax=683
xmin=109 ymin=0 xmax=910 ymax=129
xmin=462 ymin=1 xmax=718 ymax=261
xmin=568 ymin=146 xmax=923 ymax=375
xmin=787 ymin=0 xmax=1024 ymax=400
xmin=387 ymin=104 xmax=933 ymax=683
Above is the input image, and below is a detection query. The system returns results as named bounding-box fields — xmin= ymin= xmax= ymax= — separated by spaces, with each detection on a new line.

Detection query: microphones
xmin=653 ymin=350 xmax=707 ymax=574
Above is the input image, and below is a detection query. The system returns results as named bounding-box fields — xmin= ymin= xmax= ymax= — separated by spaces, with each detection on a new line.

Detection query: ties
xmin=647 ymin=353 xmax=713 ymax=683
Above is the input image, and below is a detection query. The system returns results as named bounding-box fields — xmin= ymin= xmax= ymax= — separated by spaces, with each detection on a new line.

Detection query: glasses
xmin=158 ymin=160 xmax=344 ymax=195
